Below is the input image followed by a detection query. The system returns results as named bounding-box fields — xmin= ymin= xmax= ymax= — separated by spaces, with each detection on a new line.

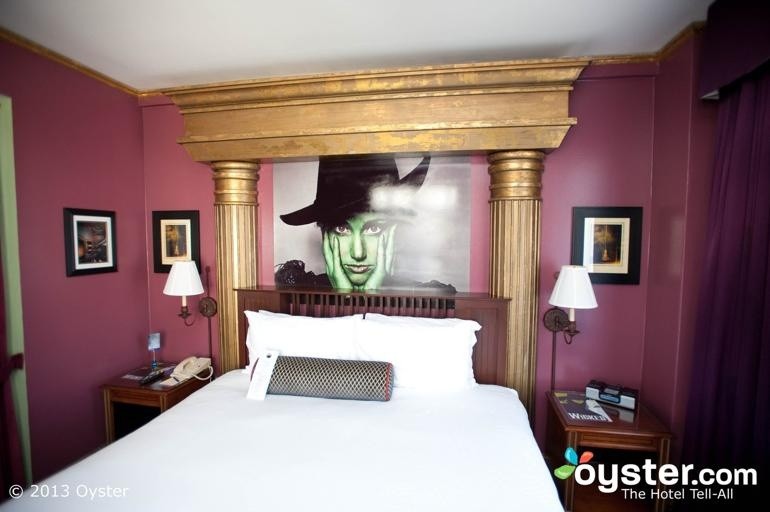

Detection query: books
xmin=552 ymin=390 xmax=613 ymax=428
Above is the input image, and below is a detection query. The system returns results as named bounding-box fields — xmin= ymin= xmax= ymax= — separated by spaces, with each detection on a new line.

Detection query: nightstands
xmin=545 ymin=391 xmax=672 ymax=512
xmin=100 ymin=366 xmax=210 ymax=446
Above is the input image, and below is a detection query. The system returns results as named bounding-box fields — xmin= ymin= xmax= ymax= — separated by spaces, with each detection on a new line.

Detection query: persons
xmin=274 ymin=155 xmax=459 ymax=295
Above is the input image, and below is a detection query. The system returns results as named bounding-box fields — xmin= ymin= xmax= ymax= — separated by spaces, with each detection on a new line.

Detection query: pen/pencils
xmin=173 ymin=377 xmax=180 ymax=383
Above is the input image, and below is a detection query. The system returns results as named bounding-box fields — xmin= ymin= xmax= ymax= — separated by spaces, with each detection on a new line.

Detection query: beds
xmin=0 ymin=285 xmax=565 ymax=512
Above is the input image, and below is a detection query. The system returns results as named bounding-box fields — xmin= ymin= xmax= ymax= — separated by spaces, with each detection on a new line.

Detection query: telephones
xmin=170 ymin=356 xmax=213 ymax=381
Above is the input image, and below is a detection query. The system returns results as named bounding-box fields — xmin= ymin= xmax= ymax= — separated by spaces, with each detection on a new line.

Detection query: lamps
xmin=163 ymin=261 xmax=217 ymax=366
xmin=544 ymin=265 xmax=598 ymax=390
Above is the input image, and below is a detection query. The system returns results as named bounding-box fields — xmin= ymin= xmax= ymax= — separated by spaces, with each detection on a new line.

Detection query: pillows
xmin=244 ymin=310 xmax=482 ymax=401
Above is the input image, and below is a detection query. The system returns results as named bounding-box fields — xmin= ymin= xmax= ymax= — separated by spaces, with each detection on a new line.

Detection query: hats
xmin=280 ymin=155 xmax=431 ymax=227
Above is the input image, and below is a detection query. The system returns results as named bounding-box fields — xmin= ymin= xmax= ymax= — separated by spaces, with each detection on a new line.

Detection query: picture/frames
xmin=152 ymin=210 xmax=201 ymax=273
xmin=572 ymin=207 xmax=642 ymax=284
xmin=63 ymin=208 xmax=118 ymax=277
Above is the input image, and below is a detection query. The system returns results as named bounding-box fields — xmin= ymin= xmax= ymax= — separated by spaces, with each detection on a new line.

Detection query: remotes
xmin=140 ymin=370 xmax=164 ymax=385
